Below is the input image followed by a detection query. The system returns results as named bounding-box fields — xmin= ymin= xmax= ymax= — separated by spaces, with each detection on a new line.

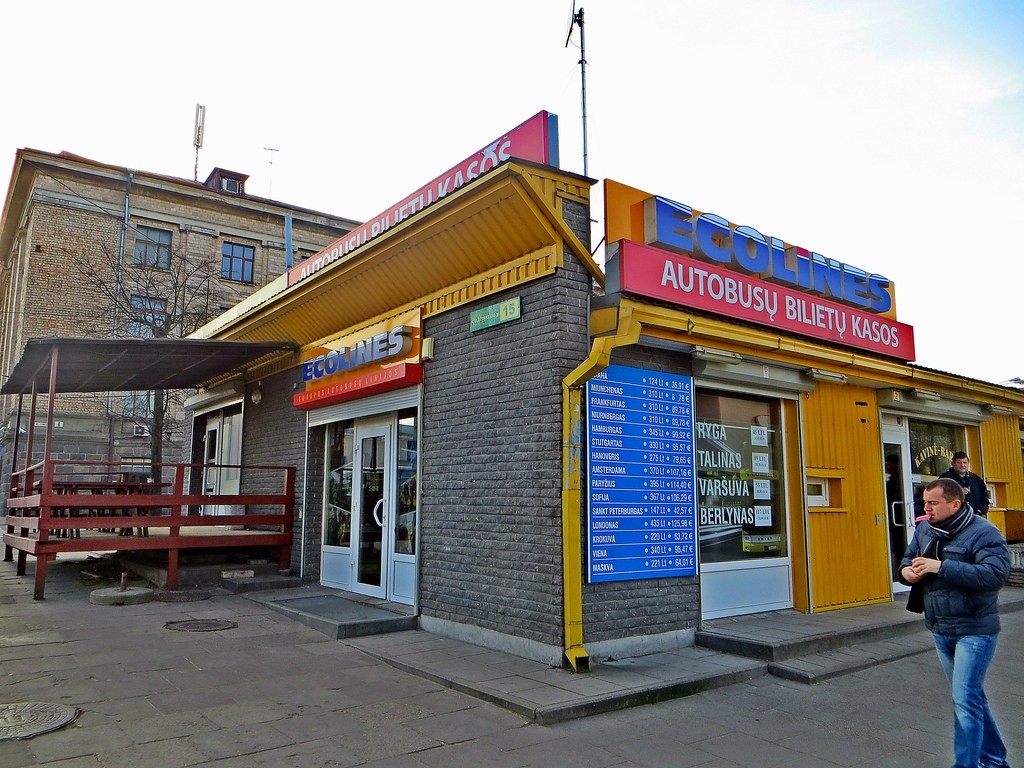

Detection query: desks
xmin=33 ymin=480 xmax=172 ymax=539
xmin=12 ymin=487 xmax=94 ymax=539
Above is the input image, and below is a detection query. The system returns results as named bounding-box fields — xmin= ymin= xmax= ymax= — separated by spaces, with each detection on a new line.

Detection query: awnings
xmin=0 ymin=336 xmax=295 ymax=395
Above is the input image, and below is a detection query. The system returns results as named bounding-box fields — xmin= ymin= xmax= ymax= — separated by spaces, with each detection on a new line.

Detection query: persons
xmin=897 ymin=479 xmax=1010 ymax=768
xmin=940 ymin=452 xmax=989 ymax=520
